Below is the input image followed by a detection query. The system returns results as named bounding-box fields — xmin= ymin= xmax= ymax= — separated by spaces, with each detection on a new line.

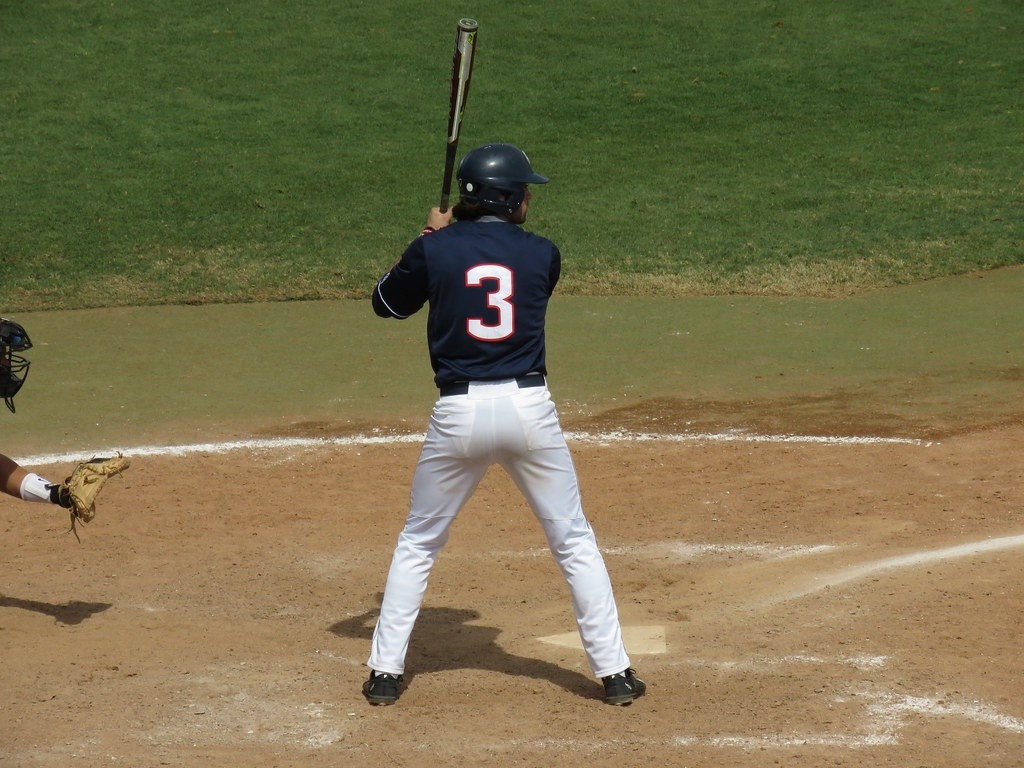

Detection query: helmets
xmin=456 ymin=143 xmax=549 ymax=216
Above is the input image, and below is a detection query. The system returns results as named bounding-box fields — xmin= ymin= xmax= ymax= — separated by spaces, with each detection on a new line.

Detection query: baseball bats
xmin=439 ymin=17 xmax=479 ymax=214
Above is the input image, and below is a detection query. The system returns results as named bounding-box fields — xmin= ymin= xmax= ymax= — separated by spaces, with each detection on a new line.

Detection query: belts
xmin=440 ymin=372 xmax=546 ymax=397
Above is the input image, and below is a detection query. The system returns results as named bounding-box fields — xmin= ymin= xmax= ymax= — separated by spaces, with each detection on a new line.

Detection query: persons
xmin=0 ymin=319 xmax=130 ymax=523
xmin=362 ymin=143 xmax=646 ymax=707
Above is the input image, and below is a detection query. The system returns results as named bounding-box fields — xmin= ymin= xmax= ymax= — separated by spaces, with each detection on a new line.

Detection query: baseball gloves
xmin=43 ymin=450 xmax=131 ymax=545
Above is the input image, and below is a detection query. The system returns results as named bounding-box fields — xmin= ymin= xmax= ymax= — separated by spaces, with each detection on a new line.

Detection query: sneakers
xmin=363 ymin=668 xmax=400 ymax=704
xmin=601 ymin=668 xmax=647 ymax=705
